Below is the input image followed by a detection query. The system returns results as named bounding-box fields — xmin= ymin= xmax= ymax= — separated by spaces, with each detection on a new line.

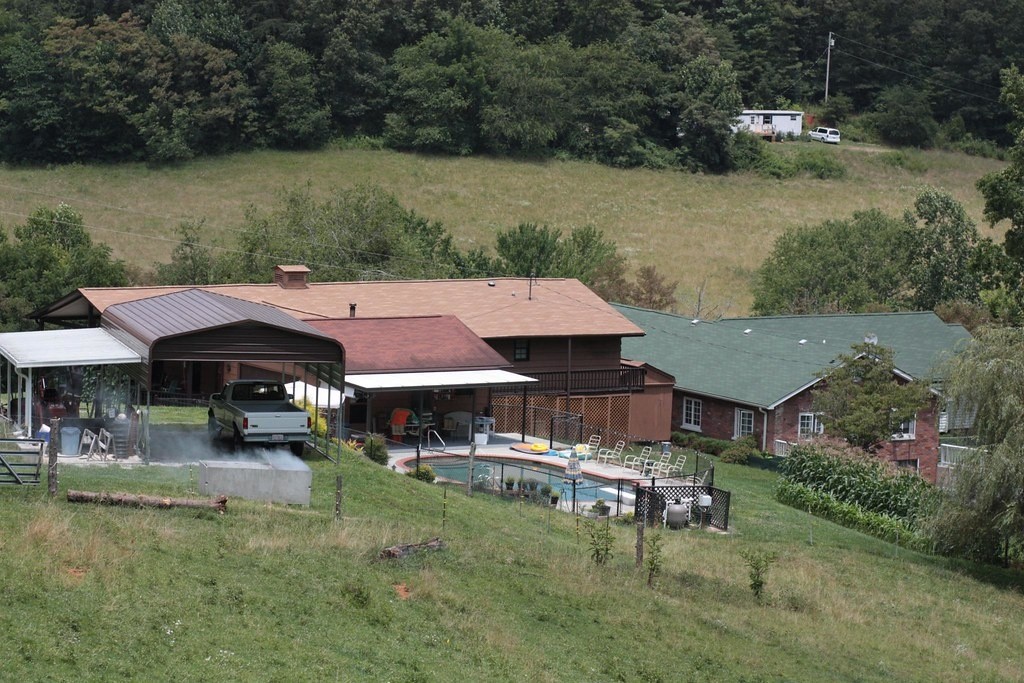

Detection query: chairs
xmin=624 ymin=446 xmax=652 ymax=475
xmin=642 ymin=451 xmax=671 ymax=481
xmin=571 ymin=435 xmax=601 ymax=463
xmin=657 ymin=455 xmax=686 ymax=483
xmin=597 ymin=441 xmax=625 ymax=468
xmin=441 ymin=417 xmax=460 ymax=441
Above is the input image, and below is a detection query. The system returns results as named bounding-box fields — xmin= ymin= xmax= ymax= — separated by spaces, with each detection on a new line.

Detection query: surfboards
xmin=508 ymin=441 xmax=546 ymax=455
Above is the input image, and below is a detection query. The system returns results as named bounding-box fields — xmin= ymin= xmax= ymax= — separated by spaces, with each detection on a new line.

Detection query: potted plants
xmin=517 ymin=476 xmax=527 ymax=492
xmin=528 ymin=478 xmax=538 ymax=490
xmin=505 ymin=475 xmax=514 ymax=490
xmin=591 ymin=498 xmax=611 ymax=516
xmin=551 ymin=491 xmax=560 ymax=504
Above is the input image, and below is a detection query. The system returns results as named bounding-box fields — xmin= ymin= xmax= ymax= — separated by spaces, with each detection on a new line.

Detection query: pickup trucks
xmin=208 ymin=380 xmax=313 ymax=457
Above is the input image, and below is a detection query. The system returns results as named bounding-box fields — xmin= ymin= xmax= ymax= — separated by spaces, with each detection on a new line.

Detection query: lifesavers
xmin=530 ymin=444 xmax=548 ymax=450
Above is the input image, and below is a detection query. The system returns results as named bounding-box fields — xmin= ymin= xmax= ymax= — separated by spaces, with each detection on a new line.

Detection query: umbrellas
xmin=564 ymin=449 xmax=583 ymax=512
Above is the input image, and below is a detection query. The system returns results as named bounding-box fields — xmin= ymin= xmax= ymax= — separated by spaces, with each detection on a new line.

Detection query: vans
xmin=808 ymin=127 xmax=841 ymax=144
